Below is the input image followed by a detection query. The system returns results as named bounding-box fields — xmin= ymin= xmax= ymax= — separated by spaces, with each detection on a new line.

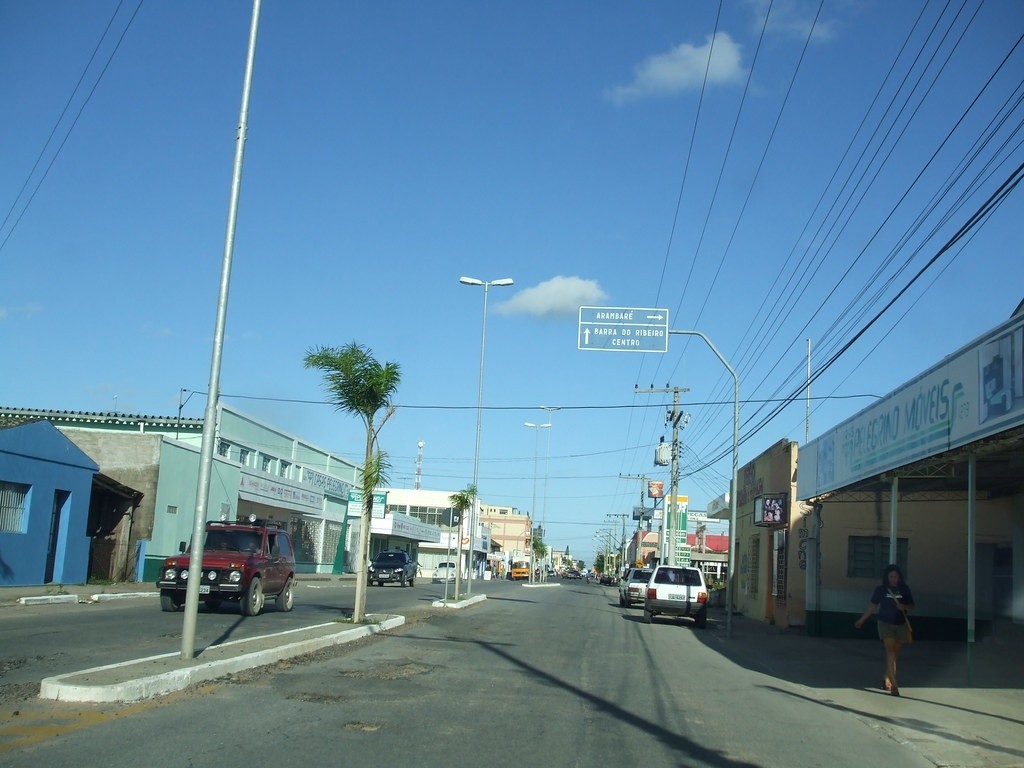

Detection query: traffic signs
xmin=578 ymin=305 xmax=668 ymax=353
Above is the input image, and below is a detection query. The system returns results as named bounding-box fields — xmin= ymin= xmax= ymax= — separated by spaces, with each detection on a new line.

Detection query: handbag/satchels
xmin=905 ymin=620 xmax=912 ymax=643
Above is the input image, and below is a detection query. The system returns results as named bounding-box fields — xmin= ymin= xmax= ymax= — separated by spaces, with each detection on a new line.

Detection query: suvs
xmin=154 ymin=513 xmax=298 ymax=617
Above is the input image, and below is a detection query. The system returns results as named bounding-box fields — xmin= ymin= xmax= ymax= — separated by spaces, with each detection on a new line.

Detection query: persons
xmin=493 ymin=565 xmax=497 ymax=579
xmin=854 ymin=564 xmax=915 ymax=697
xmin=764 ymin=498 xmax=783 ymax=522
xmin=500 ymin=565 xmax=506 ymax=580
xmin=586 ymin=571 xmax=591 ymax=581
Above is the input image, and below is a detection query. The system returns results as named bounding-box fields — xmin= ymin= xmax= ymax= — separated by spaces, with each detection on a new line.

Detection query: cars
xmin=571 ymin=570 xmax=582 ymax=579
xmin=547 ymin=571 xmax=555 ymax=577
xmin=599 ymin=575 xmax=616 ymax=586
xmin=368 ymin=551 xmax=418 ymax=588
xmin=619 ymin=568 xmax=653 ymax=608
xmin=432 ymin=561 xmax=463 ymax=584
xmin=561 ymin=571 xmax=576 ymax=579
xmin=643 ymin=565 xmax=710 ymax=625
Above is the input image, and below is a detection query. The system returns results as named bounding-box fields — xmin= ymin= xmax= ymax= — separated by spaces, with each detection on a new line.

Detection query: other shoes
xmin=891 ymin=685 xmax=899 ymax=696
xmin=883 ymin=677 xmax=892 ymax=690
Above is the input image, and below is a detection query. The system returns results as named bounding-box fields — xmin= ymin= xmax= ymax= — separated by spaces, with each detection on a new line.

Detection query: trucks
xmin=511 ymin=556 xmax=538 ymax=581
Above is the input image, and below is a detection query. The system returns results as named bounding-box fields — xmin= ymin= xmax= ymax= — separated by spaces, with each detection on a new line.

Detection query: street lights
xmin=461 ymin=276 xmax=514 ymax=593
xmin=524 ymin=422 xmax=552 ymax=582
xmin=592 ymin=528 xmax=618 ymax=578
xmin=540 ymin=406 xmax=562 ymax=582
